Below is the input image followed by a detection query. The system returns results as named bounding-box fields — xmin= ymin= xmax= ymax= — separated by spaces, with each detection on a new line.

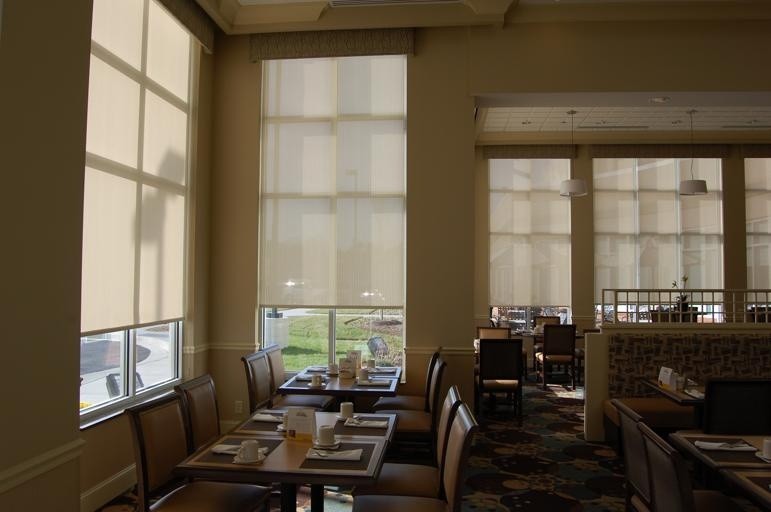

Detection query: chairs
xmin=474 ymin=326 xmax=511 ymax=374
xmin=475 ymin=338 xmax=523 ymax=427
xmin=375 ymin=356 xmax=447 ymax=465
xmin=533 ymin=316 xmax=560 ymax=371
xmin=173 ymin=374 xmax=281 ymax=496
xmin=373 ymin=352 xmax=440 ymax=448
xmin=602 ymin=396 xmax=693 ymax=439
xmin=260 ymin=348 xmax=336 ymax=410
xmin=535 ymin=324 xmax=576 ymax=391
xmin=636 ymin=421 xmax=694 ymax=511
xmin=611 ymin=399 xmax=747 ymax=512
xmin=241 ymin=351 xmax=323 ymax=416
xmin=125 ymin=393 xmax=272 ymax=511
xmin=351 ymin=383 xmax=462 ymax=500
xmin=352 ymin=403 xmax=478 ymax=512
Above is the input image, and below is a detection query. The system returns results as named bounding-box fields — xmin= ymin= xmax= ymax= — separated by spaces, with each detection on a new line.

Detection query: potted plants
xmin=673 ymin=275 xmax=692 ymax=312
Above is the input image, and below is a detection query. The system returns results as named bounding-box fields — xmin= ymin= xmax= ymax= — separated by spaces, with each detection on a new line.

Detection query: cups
xmin=312 ymin=376 xmax=322 ymax=385
xmin=763 ymin=438 xmax=771 ymax=459
xmin=340 ymin=402 xmax=353 ymax=418
xmin=320 ymin=425 xmax=334 ymax=443
xmin=359 ymin=370 xmax=368 ymax=379
xmin=367 ymin=360 xmax=375 ymax=368
xmin=238 ymin=440 xmax=258 ymax=460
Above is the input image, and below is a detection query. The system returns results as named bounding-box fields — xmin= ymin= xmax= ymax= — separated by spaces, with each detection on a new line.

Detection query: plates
xmin=212 ymin=445 xmax=268 ymax=457
xmin=344 ymin=419 xmax=388 ymax=427
xmin=695 ymin=441 xmax=756 ymax=450
xmin=305 ymin=448 xmax=362 ymax=460
xmin=233 ymin=455 xmax=265 ymax=462
xmin=253 ymin=414 xmax=284 ymax=422
xmin=312 ymin=437 xmax=340 ymax=445
xmin=308 ymin=383 xmax=326 ymax=387
xmin=335 ymin=413 xmax=356 ymax=420
xmin=755 ymin=452 xmax=771 ymax=463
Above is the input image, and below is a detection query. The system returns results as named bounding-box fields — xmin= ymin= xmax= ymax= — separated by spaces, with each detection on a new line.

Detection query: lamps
xmin=648 ymin=96 xmax=671 ymax=105
xmin=679 ymin=109 xmax=708 ymax=196
xmin=560 ymin=110 xmax=588 ymax=198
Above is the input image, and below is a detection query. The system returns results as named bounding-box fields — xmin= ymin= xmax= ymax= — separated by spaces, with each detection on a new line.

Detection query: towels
xmin=253 ymin=412 xmax=285 ymax=422
xmin=695 ymin=440 xmax=759 ymax=450
xmin=685 ymin=388 xmax=705 ymax=399
xmin=307 ymin=366 xmax=328 ymax=372
xmin=370 ymin=366 xmax=397 ymax=373
xmin=308 ymin=447 xmax=364 ymax=460
xmin=296 ymin=373 xmax=326 ymax=381
xmin=357 ymin=379 xmax=392 ymax=385
xmin=345 ymin=417 xmax=388 ymax=428
xmin=212 ymin=443 xmax=270 ymax=455
